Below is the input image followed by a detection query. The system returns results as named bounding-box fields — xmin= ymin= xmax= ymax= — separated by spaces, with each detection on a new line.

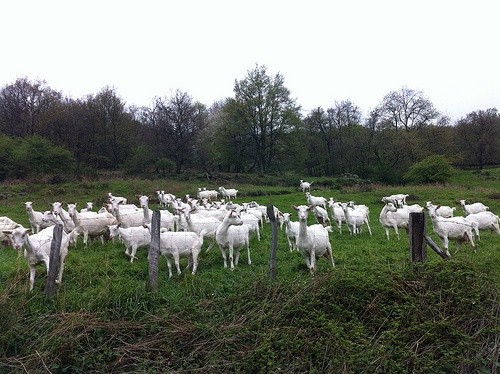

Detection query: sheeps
xmin=0 ymin=180 xmax=500 ymax=296
